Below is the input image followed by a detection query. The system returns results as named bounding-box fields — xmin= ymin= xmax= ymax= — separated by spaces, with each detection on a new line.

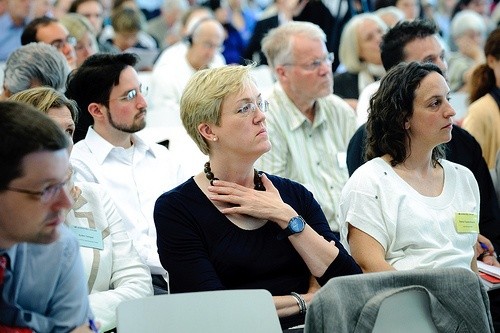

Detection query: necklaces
xmin=203 ymin=162 xmax=266 ymax=207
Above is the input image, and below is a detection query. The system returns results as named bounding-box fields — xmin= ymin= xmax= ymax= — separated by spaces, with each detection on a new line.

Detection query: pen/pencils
xmin=480 ymin=242 xmax=488 ymax=249
xmin=88 ymin=317 xmax=98 ymax=333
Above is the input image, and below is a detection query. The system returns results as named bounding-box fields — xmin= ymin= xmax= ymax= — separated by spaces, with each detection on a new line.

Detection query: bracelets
xmin=288 ymin=291 xmax=308 ymax=316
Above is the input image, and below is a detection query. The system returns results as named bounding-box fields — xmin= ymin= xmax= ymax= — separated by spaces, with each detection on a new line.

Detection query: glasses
xmin=7 ymin=179 xmax=75 ymax=200
xmin=218 ymin=100 xmax=269 ymax=116
xmin=281 ymin=52 xmax=335 ymax=70
xmin=194 ymin=40 xmax=225 ymax=52
xmin=107 ymin=84 xmax=149 ymax=103
xmin=52 ymin=37 xmax=77 ymax=48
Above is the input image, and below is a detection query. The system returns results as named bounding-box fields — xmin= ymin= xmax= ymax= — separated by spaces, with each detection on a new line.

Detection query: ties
xmin=0 ymin=256 xmax=32 ymax=333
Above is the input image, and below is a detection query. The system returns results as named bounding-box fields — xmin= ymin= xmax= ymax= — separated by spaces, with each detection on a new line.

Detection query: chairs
xmin=304 ymin=267 xmax=495 ymax=333
xmin=116 ymin=289 xmax=283 ymax=333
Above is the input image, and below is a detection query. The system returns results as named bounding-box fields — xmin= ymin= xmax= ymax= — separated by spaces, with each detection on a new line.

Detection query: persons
xmin=1 ymin=0 xmax=500 ymax=172
xmin=65 ymin=52 xmax=190 ymax=296
xmin=253 ymin=21 xmax=363 ymax=255
xmin=346 ymin=19 xmax=500 ymax=266
xmin=4 ymin=41 xmax=71 ymax=94
xmin=0 ymin=101 xmax=94 ymax=333
xmin=0 ymin=88 xmax=153 ymax=333
xmin=338 ymin=61 xmax=481 ymax=275
xmin=153 ymin=61 xmax=363 ymax=318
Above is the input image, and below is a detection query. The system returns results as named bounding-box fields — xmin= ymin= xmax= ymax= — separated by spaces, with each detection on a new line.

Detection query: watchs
xmin=283 ymin=215 xmax=307 ymax=237
